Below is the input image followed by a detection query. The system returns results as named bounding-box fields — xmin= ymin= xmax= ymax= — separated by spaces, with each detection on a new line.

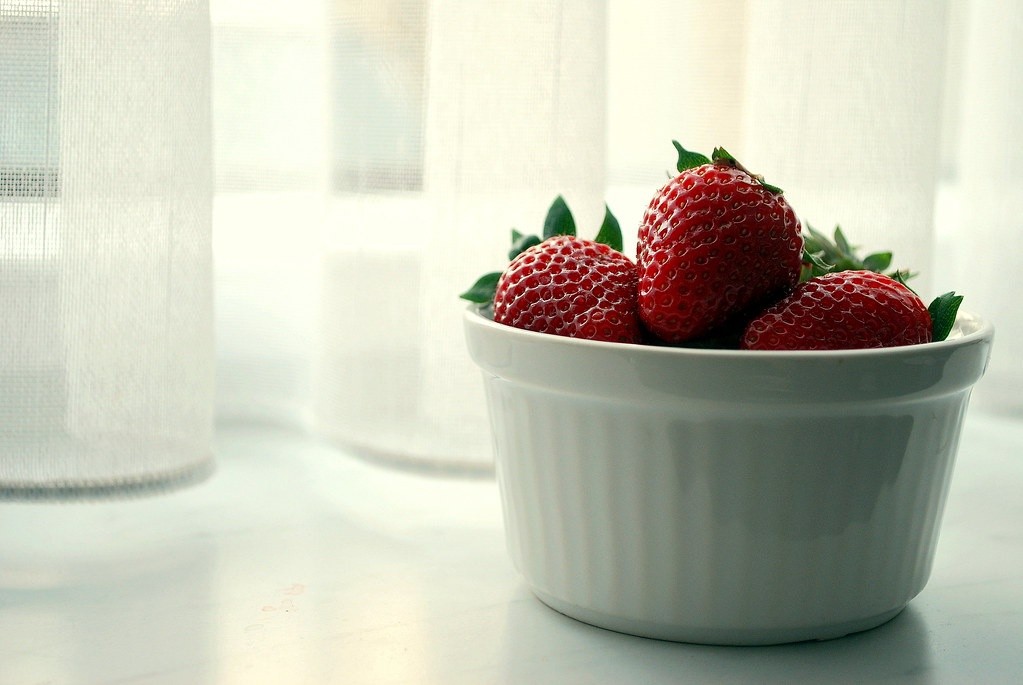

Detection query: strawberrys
xmin=457 ymin=139 xmax=963 ymax=350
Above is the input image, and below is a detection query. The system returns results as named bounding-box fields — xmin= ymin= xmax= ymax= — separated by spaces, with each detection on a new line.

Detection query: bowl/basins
xmin=461 ymin=303 xmax=996 ymax=646
